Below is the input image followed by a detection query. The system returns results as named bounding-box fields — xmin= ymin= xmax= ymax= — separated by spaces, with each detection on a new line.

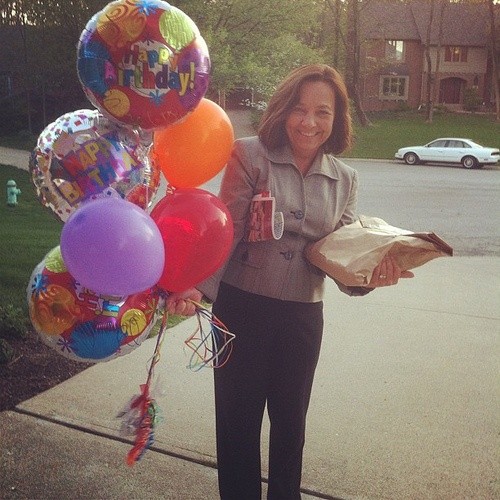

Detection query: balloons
xmin=147 ymin=188 xmax=234 ymax=293
xmin=151 ymin=97 xmax=235 ymax=191
xmin=76 ymin=0 xmax=212 ymax=133
xmin=29 ymin=109 xmax=145 ymax=226
xmin=25 ymin=244 xmax=161 ymax=365
xmin=59 ymin=197 xmax=166 ymax=299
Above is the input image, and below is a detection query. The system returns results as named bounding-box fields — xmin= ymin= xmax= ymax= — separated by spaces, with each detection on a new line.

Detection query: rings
xmin=380 ymin=274 xmax=387 ymax=278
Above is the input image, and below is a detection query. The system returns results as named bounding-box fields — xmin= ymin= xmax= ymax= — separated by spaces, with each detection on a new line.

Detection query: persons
xmin=165 ymin=64 xmax=415 ymax=500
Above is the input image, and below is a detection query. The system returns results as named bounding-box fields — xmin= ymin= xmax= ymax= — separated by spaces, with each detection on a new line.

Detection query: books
xmin=238 ymin=190 xmax=284 ymax=243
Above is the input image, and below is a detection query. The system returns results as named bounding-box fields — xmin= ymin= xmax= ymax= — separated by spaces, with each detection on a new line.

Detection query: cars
xmin=394 ymin=137 xmax=500 ymax=170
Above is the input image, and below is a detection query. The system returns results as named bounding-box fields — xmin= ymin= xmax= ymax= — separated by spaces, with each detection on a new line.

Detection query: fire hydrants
xmin=6 ymin=179 xmax=22 ymax=208
